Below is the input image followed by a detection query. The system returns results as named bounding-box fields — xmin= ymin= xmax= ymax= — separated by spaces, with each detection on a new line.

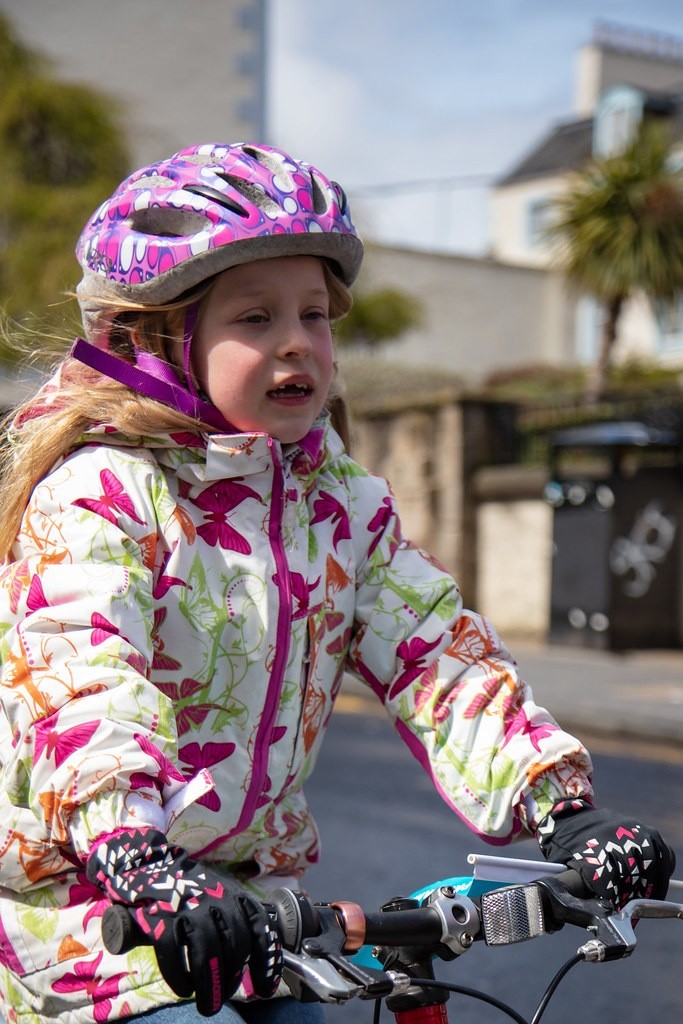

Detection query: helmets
xmin=75 ymin=143 xmax=363 ymax=304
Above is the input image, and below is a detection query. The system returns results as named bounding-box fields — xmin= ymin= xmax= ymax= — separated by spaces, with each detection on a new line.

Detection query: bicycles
xmin=101 ymin=869 xmax=683 ymax=1024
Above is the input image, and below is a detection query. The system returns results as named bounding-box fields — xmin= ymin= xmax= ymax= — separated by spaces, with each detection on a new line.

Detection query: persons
xmin=0 ymin=141 xmax=678 ymax=1024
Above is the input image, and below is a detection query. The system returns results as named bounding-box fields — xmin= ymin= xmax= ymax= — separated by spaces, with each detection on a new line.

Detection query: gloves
xmin=85 ymin=829 xmax=283 ymax=1019
xmin=535 ymin=800 xmax=677 ymax=928
